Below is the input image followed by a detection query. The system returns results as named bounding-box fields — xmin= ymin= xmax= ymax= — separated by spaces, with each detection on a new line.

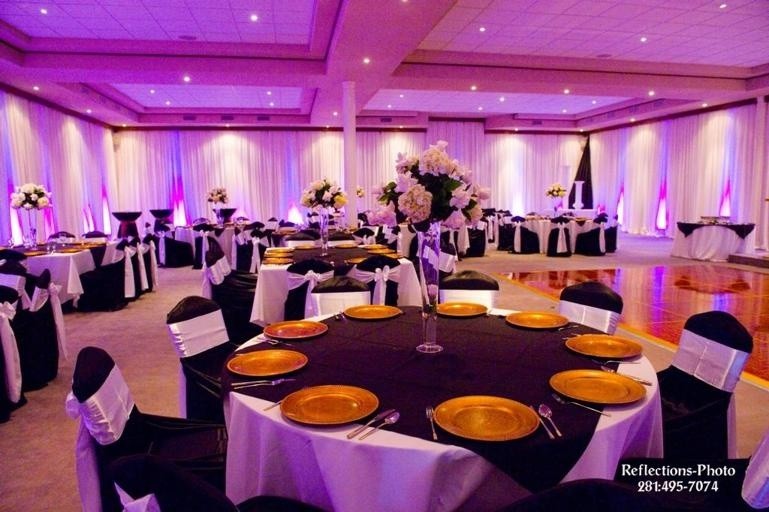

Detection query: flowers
xmin=207 ymin=188 xmax=228 ymax=205
xmin=545 ymin=183 xmax=568 ymax=198
xmin=10 ymin=183 xmax=54 ymax=210
xmin=300 ymin=179 xmax=347 ymax=207
xmin=366 ymin=141 xmax=493 ymax=230
xmin=356 ymin=183 xmax=366 ymax=199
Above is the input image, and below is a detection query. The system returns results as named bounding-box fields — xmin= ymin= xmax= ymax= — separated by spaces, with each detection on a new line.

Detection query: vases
xmin=553 ymin=197 xmax=559 ymax=218
xmin=318 ymin=206 xmax=329 ymax=258
xmin=213 ymin=202 xmax=223 ymax=228
xmin=409 ymin=221 xmax=445 ymax=353
xmin=24 ymin=208 xmax=38 ymax=250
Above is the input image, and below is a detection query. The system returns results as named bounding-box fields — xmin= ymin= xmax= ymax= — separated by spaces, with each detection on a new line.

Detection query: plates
xmin=296 ymin=246 xmax=317 ymax=250
xmin=265 ymin=258 xmax=295 ymax=264
xmin=227 ymin=349 xmax=308 ymax=376
xmin=505 ymin=311 xmax=569 ymax=328
xmin=364 ymin=246 xmax=388 ymax=250
xmin=336 ymin=244 xmax=356 ymax=248
xmin=280 ymin=385 xmax=379 ymax=424
xmin=565 ymin=334 xmax=644 ymax=358
xmin=64 ymin=248 xmax=80 ymax=253
xmin=267 ymin=249 xmax=294 ymax=253
xmin=549 ymin=369 xmax=647 ymax=404
xmin=344 ymin=305 xmax=400 ymax=319
xmin=266 ymin=253 xmax=294 ymax=257
xmin=23 ymin=251 xmax=45 ymax=256
xmin=263 ymin=320 xmax=328 ymax=340
xmin=437 ymin=302 xmax=488 ymax=316
xmin=434 ymin=395 xmax=540 ymax=441
xmin=367 ymin=249 xmax=395 ymax=254
xmin=347 ymin=258 xmax=368 ymax=263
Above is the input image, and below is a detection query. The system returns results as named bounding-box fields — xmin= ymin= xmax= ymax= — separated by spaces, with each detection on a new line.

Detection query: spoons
xmin=538 ymin=404 xmax=562 ymax=437
xmin=359 ymin=412 xmax=401 ymax=441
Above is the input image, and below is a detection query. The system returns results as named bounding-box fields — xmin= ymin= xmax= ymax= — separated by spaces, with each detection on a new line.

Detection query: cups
xmin=59 ymin=233 xmax=66 ymax=246
xmin=43 ymin=240 xmax=50 ymax=254
xmin=50 ymin=237 xmax=57 ymax=252
xmin=79 ymin=233 xmax=86 ymax=246
xmin=7 ymin=237 xmax=14 ymax=249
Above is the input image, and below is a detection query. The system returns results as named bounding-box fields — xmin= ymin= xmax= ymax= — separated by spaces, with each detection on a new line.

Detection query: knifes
xmin=529 ymin=405 xmax=555 ymax=439
xmin=231 ymin=378 xmax=298 ymax=386
xmin=347 ymin=408 xmax=396 ymax=439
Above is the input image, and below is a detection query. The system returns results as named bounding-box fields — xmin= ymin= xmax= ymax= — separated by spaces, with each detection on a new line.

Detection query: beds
xmin=222 ymin=306 xmax=659 ymax=512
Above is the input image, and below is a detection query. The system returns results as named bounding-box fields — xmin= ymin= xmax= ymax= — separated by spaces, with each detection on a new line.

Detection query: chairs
xmin=141 ymin=233 xmax=155 ymax=292
xmin=233 ymin=226 xmax=253 ymax=270
xmin=482 ymin=211 xmax=496 ymax=243
xmin=193 ymin=224 xmax=224 ymax=269
xmin=615 ymin=432 xmax=769 ymax=512
xmin=283 ymin=259 xmax=337 ymax=321
xmin=207 ymin=236 xmax=258 ymax=282
xmin=439 ymin=268 xmax=501 ymax=309
xmin=559 ymin=282 xmax=623 ymax=334
xmin=546 ymin=215 xmax=574 ymax=258
xmin=205 ymin=250 xmax=256 ymax=300
xmin=312 ymin=275 xmax=372 ymax=316
xmin=346 ymin=255 xmax=408 ymax=306
xmin=606 ymin=215 xmax=619 ymax=252
xmin=250 ymin=229 xmax=272 ymax=273
xmin=511 ymin=216 xmax=539 ymax=253
xmin=154 ymin=219 xmax=193 ymax=268
xmin=350 ymin=228 xmax=377 ymax=245
xmin=469 ymin=218 xmax=487 ymax=257
xmin=0 ymin=285 xmax=27 ymax=423
xmin=66 ymin=346 xmax=227 ymax=511
xmin=129 ymin=237 xmax=141 ymax=302
xmin=577 ymin=215 xmax=609 ymax=257
xmin=79 ymin=238 xmax=130 ymax=310
xmin=656 ymin=310 xmax=753 ymax=458
xmin=497 ymin=214 xmax=513 ymax=250
xmin=166 ymin=296 xmax=240 ymax=419
xmin=439 ymin=241 xmax=457 ymax=279
xmin=13 ymin=269 xmax=58 ymax=391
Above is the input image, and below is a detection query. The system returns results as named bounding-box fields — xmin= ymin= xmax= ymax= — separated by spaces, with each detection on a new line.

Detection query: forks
xmin=426 ymin=406 xmax=438 ymax=440
xmin=552 ymin=393 xmax=612 ymax=417
xmin=591 ymin=359 xmax=641 ymax=366
